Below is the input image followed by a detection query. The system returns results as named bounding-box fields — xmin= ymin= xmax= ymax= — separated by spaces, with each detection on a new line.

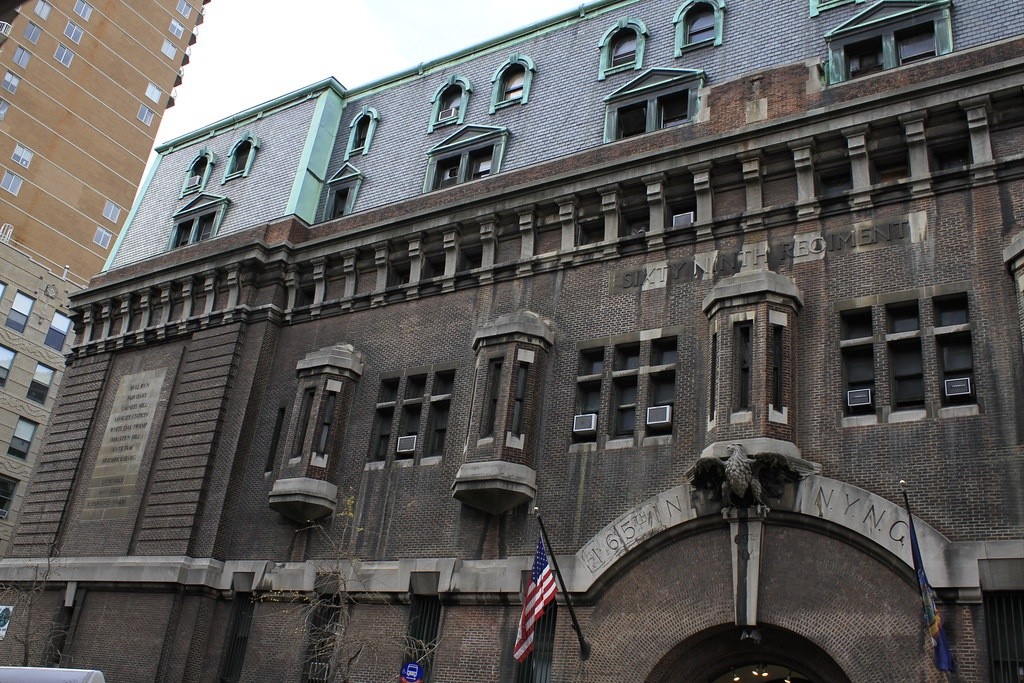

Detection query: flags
xmin=513 ymin=532 xmax=558 ymax=663
xmin=902 ymin=490 xmax=955 ymax=674
